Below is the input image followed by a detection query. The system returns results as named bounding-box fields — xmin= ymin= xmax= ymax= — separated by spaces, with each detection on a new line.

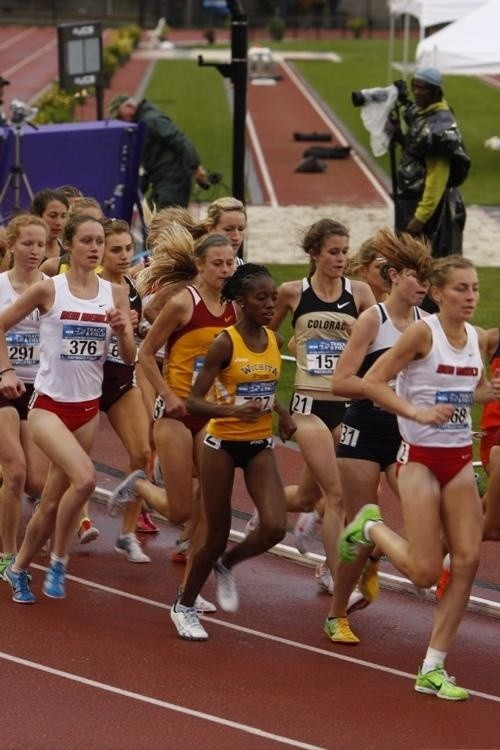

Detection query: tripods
xmin=0 ymin=128 xmax=34 ymax=217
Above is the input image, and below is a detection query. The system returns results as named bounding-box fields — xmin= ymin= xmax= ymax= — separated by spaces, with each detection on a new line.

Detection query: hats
xmin=108 ymin=94 xmax=130 ymax=120
xmin=414 ymin=67 xmax=443 ymax=86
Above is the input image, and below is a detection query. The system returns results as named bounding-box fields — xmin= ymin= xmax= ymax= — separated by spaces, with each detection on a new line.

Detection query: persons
xmin=380 ymin=63 xmax=472 ymax=318
xmin=108 ymin=93 xmax=212 ymax=212
xmin=245 ymin=219 xmax=499 ymax=701
xmin=1 ymin=185 xmax=299 ymax=639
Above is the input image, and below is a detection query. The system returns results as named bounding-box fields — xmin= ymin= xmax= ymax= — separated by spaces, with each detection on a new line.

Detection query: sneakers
xmin=294 ymin=504 xmax=453 ymax=614
xmin=43 ymin=560 xmax=66 ymax=598
xmin=214 ymin=558 xmax=239 ymax=613
xmin=77 ymin=520 xmax=99 ymax=543
xmin=415 ymin=664 xmax=468 ymax=701
xmin=178 ymin=585 xmax=217 ymax=612
xmin=4 ymin=566 xmax=37 ymax=603
xmin=170 ymin=601 xmax=207 ymax=640
xmin=105 ymin=456 xmax=259 ymax=564
xmin=0 ymin=553 xmax=32 ymax=582
xmin=324 ymin=617 xmax=359 ymax=644
